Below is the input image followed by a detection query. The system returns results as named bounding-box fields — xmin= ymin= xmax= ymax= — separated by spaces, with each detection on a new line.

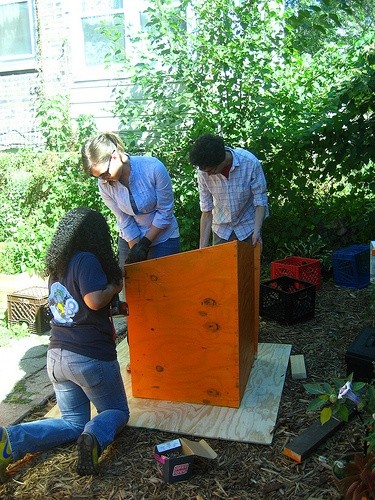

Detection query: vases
xmin=332 ymin=452 xmax=375 ymax=493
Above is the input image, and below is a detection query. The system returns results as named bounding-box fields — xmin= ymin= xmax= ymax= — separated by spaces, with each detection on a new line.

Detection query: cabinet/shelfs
xmin=124 ymin=242 xmax=258 ymax=410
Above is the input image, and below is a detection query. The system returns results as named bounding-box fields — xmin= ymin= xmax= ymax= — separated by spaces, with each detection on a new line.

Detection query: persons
xmin=189 ymin=133 xmax=269 ymax=252
xmin=0 ymin=207 xmax=130 ymax=484
xmin=81 ymin=133 xmax=181 ymax=373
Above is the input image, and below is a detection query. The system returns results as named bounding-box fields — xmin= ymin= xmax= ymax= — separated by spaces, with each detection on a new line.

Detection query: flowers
xmin=303 ymin=372 xmax=375 ymax=469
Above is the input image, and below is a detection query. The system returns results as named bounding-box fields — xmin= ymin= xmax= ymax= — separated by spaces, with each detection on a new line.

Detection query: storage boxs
xmin=345 ymin=326 xmax=375 ymax=385
xmin=7 ymin=286 xmax=52 ymax=335
xmin=332 ymin=245 xmax=372 ymax=289
xmin=152 ymin=437 xmax=218 ymax=484
xmin=271 ymin=256 xmax=320 ymax=292
xmin=259 ymin=276 xmax=316 ymax=326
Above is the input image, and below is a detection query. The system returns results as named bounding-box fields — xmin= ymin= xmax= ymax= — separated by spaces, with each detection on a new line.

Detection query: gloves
xmin=123 ymin=236 xmax=152 ymax=264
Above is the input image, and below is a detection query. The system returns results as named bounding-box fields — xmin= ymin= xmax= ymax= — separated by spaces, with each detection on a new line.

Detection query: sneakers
xmin=0 ymin=425 xmax=14 ymax=485
xmin=75 ymin=432 xmax=98 ymax=476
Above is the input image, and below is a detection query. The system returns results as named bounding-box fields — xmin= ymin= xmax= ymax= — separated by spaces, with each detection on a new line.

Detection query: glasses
xmin=204 ymin=166 xmax=217 ymax=175
xmin=93 ymin=150 xmax=115 ymax=181
